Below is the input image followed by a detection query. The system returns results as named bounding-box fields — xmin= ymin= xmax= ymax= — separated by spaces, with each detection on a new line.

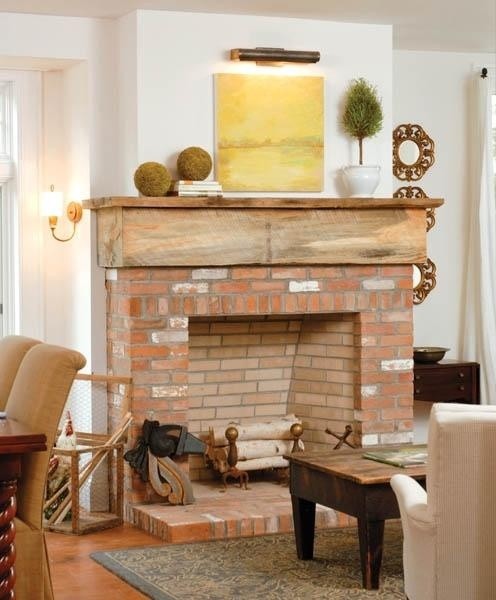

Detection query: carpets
xmin=89 ymin=521 xmax=408 ymax=600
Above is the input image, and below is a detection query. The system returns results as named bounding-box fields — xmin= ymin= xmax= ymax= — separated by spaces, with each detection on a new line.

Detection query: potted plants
xmin=339 ymin=76 xmax=386 ymax=198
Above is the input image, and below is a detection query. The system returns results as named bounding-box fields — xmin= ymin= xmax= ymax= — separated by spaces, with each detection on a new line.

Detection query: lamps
xmin=230 ymin=45 xmax=320 ymax=67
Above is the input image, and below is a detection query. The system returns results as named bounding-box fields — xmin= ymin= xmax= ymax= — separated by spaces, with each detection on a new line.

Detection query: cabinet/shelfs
xmin=414 ymin=357 xmax=480 ymax=404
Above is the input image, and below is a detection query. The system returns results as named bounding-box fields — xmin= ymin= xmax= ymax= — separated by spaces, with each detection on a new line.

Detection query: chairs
xmin=390 ymin=402 xmax=496 ymax=600
xmin=0 ymin=333 xmax=86 ymax=600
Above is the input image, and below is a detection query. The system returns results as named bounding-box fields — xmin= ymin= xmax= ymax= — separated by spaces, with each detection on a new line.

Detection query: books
xmin=171 ymin=179 xmax=223 ymax=198
xmin=364 ymin=449 xmax=429 ymax=468
